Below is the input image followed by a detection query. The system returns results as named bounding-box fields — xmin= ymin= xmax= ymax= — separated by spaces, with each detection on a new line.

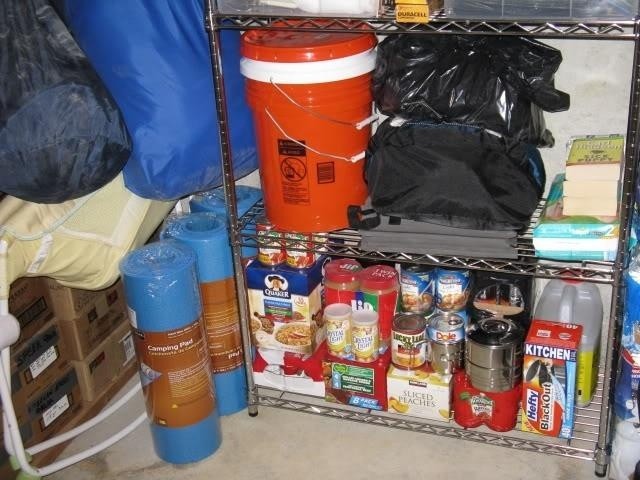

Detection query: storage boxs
xmin=6 ymin=318 xmax=74 ymax=419
xmin=17 ymin=363 xmax=81 ymax=451
xmin=68 ymin=319 xmax=136 ymax=404
xmin=45 ymin=273 xmax=129 ymax=363
xmin=7 ymin=275 xmax=56 ymax=360
xmin=394 ymin=0 xmax=444 ymax=23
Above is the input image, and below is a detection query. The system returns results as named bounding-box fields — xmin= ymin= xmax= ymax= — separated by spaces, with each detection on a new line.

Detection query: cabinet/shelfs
xmin=201 ymin=0 xmax=640 ymax=477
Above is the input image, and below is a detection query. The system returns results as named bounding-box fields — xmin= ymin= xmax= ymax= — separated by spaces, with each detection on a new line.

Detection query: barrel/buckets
xmin=239 ymin=18 xmax=379 ymax=234
xmin=533 ymin=269 xmax=605 ymax=407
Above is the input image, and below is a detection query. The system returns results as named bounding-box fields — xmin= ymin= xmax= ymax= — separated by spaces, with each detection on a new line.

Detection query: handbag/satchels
xmin=364 ymin=119 xmax=547 ymax=231
xmin=373 ymin=26 xmax=562 ymax=149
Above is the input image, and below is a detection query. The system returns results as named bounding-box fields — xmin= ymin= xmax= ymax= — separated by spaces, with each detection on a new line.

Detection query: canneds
xmin=256 ymin=222 xmax=525 ymax=392
